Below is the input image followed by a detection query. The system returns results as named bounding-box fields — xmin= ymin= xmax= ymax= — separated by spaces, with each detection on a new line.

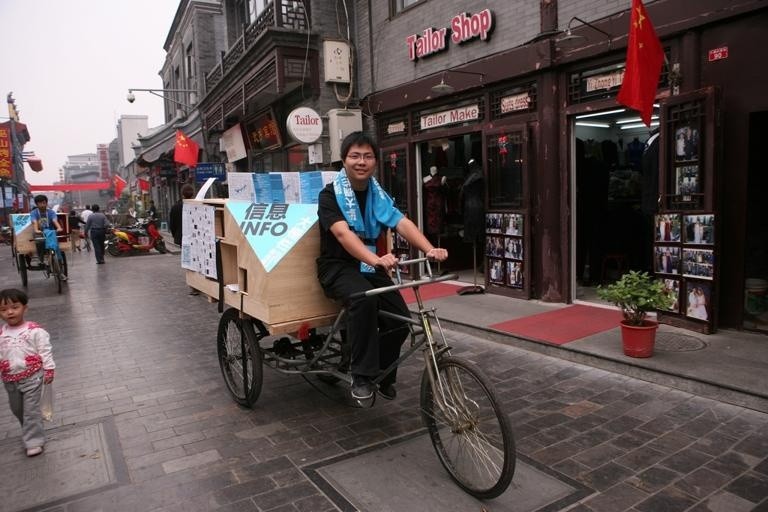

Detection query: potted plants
xmin=597 ymin=269 xmax=677 ymax=361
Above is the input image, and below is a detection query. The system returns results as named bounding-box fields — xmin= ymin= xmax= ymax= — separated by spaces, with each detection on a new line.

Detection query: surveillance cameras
xmin=127 ymin=93 xmax=137 ymax=103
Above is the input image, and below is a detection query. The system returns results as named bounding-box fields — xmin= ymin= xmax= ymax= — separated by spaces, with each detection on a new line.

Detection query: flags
xmin=175 ymin=131 xmax=200 ymax=167
xmin=616 ymin=0 xmax=664 ymax=128
xmin=115 ymin=174 xmax=127 ymax=199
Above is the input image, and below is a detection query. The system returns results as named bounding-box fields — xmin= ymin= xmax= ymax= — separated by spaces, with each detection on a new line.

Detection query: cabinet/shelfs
xmin=184 ymin=199 xmax=344 ymax=326
xmin=7 ymin=213 xmax=69 ymax=252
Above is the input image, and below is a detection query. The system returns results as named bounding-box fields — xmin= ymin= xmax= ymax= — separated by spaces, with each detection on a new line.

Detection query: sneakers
xmin=368 ymin=375 xmax=397 ymax=401
xmin=59 ymin=273 xmax=67 ymax=281
xmin=27 ymin=446 xmax=43 ymax=457
xmin=351 ymin=375 xmax=376 ymax=410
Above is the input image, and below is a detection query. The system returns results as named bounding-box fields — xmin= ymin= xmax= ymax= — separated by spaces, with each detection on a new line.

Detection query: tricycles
xmin=217 ymin=257 xmax=516 ymax=499
xmin=18 ymin=230 xmax=67 ymax=294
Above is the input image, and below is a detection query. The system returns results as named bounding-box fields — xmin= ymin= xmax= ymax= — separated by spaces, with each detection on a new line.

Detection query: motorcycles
xmin=107 ymin=211 xmax=166 ymax=257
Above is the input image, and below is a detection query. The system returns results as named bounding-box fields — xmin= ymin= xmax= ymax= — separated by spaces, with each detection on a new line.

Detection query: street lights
xmin=126 ymin=88 xmax=201 ymax=112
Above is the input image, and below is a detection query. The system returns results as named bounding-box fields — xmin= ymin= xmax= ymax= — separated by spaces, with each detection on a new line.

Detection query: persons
xmin=84 ymin=205 xmax=110 ymax=264
xmin=0 ymin=289 xmax=56 ymax=456
xmin=169 ymin=185 xmax=199 ymax=297
xmin=510 ymin=265 xmax=520 ymax=285
xmin=317 ymin=132 xmax=448 ymax=400
xmin=110 ymin=207 xmax=118 ymax=223
xmin=687 ymin=287 xmax=708 ymax=320
xmin=81 ymin=203 xmax=93 ymax=249
xmin=146 ymin=200 xmax=156 ymax=219
xmin=68 ymin=211 xmax=86 ymax=252
xmin=694 ymin=220 xmax=704 ymax=244
xmin=662 ymin=251 xmax=672 ymax=273
xmin=676 ymin=127 xmax=693 ymax=161
xmin=659 ymin=216 xmax=672 ymax=242
xmin=507 ymin=239 xmax=521 ymax=259
xmin=491 ymin=261 xmax=503 ymax=282
xmin=31 ymin=195 xmax=69 ymax=281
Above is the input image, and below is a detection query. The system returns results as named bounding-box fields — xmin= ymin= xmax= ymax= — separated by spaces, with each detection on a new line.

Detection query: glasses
xmin=347 ymin=154 xmax=375 ymax=160
